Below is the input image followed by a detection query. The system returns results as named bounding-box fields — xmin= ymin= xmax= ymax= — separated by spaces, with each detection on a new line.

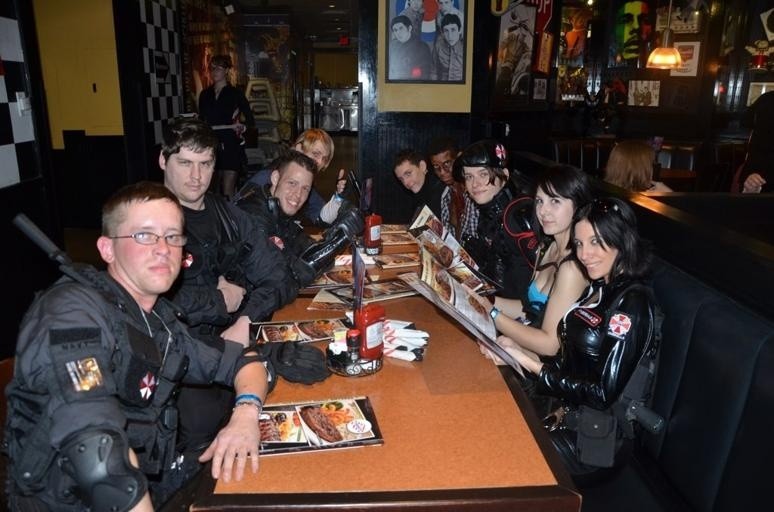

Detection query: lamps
xmin=644 ymin=0 xmax=683 ymax=71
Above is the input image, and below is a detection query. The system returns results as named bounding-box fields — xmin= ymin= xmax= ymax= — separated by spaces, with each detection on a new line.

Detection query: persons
xmin=388 ymin=16 xmax=433 ymax=81
xmin=258 ymin=37 xmax=274 ymax=76
xmin=596 ymin=80 xmax=615 ymax=129
xmin=431 ymin=14 xmax=464 ymax=81
xmin=643 ymin=87 xmax=651 ymax=106
xmin=199 ymin=52 xmax=258 ymax=202
xmin=615 ymin=2 xmax=653 ymax=60
xmin=394 ymin=128 xmax=665 ymax=508
xmin=497 ymin=18 xmax=531 ymax=94
xmin=633 ymin=88 xmax=642 ymax=106
xmin=1 ymin=128 xmax=366 ymax=510
xmin=738 ymin=91 xmax=773 ymax=194
xmin=398 ymin=1 xmax=425 ymax=38
xmin=437 ymin=1 xmax=464 ymax=32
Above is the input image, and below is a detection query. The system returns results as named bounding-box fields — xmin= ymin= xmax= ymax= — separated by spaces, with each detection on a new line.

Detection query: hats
xmin=454 ymin=139 xmax=506 ymax=167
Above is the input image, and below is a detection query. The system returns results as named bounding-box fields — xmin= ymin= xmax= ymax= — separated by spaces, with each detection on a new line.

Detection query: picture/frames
xmin=627 ymin=78 xmax=662 ymax=111
xmin=531 ymin=75 xmax=550 ymax=102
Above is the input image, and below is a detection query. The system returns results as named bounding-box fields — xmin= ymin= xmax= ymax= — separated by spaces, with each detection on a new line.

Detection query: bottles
xmin=364 ymin=215 xmax=381 ymax=246
xmin=353 ymin=303 xmax=384 ymax=360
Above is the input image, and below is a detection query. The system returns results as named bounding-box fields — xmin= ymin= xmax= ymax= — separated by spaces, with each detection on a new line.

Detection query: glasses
xmin=106 ymin=233 xmax=188 ymax=248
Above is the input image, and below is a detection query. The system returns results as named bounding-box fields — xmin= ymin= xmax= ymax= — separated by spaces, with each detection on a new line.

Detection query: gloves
xmin=334 ymin=177 xmax=351 ymax=198
